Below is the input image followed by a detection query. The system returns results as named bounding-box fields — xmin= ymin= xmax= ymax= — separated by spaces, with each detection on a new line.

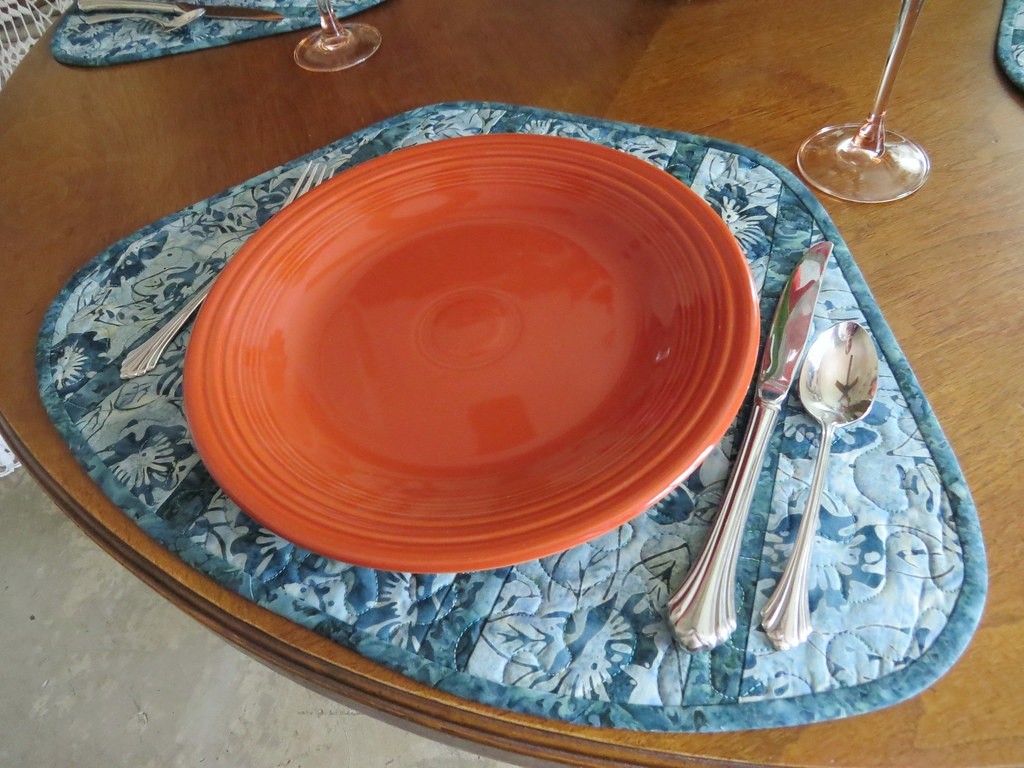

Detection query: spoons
xmin=84 ymin=8 xmax=206 ymax=32
xmin=761 ymin=320 xmax=880 ymax=651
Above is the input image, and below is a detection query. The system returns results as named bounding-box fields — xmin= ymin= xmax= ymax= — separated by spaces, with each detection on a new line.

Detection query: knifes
xmin=77 ymin=0 xmax=284 ymax=21
xmin=664 ymin=242 xmax=834 ymax=653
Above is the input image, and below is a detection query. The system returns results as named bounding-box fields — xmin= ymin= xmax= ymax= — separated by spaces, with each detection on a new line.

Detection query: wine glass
xmin=294 ymin=0 xmax=383 ymax=72
xmin=797 ymin=0 xmax=932 ymax=204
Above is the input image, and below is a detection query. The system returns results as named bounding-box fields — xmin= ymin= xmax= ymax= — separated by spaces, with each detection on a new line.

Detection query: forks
xmin=118 ymin=160 xmax=336 ymax=380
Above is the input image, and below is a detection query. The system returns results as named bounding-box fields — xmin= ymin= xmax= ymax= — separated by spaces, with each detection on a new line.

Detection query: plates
xmin=181 ymin=133 xmax=761 ymax=571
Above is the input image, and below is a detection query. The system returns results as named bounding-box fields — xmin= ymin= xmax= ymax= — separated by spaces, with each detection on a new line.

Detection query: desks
xmin=0 ymin=0 xmax=1024 ymax=768
